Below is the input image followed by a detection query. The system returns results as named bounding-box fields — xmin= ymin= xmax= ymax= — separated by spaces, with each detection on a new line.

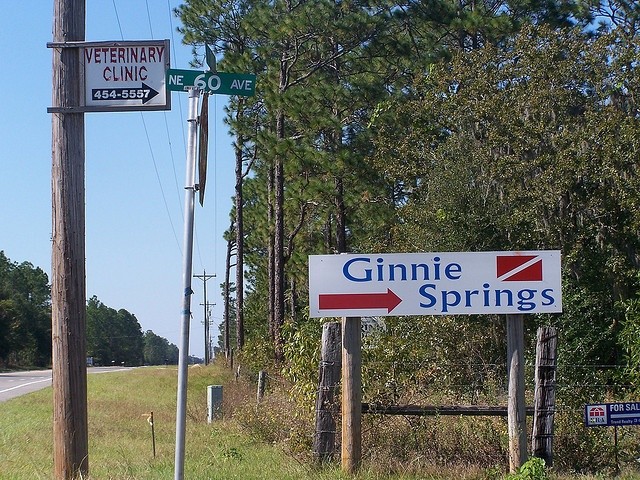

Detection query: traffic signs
xmin=80 ymin=38 xmax=169 ymax=112
xmin=585 ymin=400 xmax=640 ymax=426
xmin=309 ymin=251 xmax=563 ymax=316
xmin=166 ymin=44 xmax=257 ymax=207
